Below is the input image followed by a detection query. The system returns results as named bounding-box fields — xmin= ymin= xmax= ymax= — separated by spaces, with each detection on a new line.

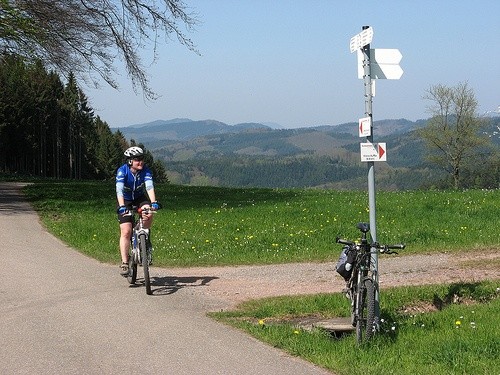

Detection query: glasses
xmin=134 ymin=156 xmax=144 ymax=161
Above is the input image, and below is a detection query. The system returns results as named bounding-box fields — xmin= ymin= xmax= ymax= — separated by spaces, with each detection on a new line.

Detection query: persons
xmin=116 ymin=146 xmax=160 ymax=275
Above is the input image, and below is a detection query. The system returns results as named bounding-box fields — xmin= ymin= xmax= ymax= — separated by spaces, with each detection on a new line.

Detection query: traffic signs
xmin=358 ymin=117 xmax=371 ymax=138
xmin=349 ymin=27 xmax=374 ymax=53
xmin=360 ymin=142 xmax=387 ymax=161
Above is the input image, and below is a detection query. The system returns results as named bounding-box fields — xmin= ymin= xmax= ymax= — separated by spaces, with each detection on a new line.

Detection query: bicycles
xmin=335 ymin=223 xmax=406 ymax=347
xmin=126 ymin=205 xmax=158 ymax=295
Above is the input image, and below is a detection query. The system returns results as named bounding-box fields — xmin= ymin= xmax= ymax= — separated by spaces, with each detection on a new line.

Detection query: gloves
xmin=119 ymin=207 xmax=129 ymax=216
xmin=150 ymin=202 xmax=159 ymax=211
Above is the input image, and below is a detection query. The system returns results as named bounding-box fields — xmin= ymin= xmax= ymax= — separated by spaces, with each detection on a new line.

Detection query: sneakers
xmin=120 ymin=263 xmax=129 ymax=274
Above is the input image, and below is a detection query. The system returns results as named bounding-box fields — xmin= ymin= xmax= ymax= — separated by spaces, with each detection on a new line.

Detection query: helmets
xmin=124 ymin=147 xmax=144 ymax=157
